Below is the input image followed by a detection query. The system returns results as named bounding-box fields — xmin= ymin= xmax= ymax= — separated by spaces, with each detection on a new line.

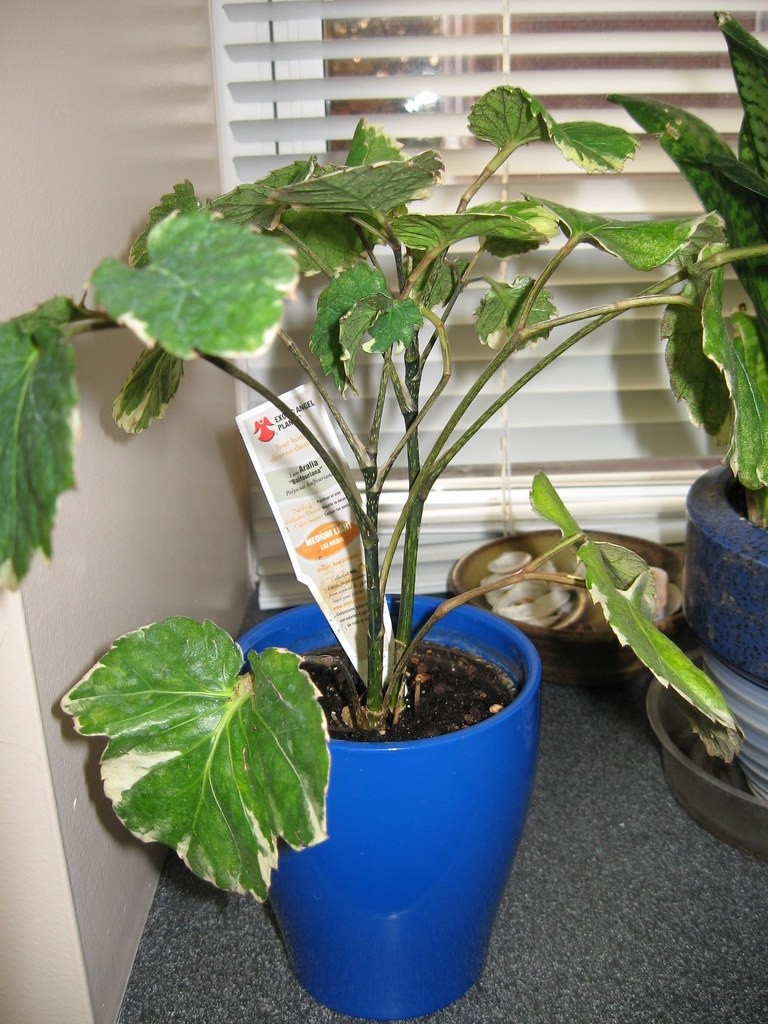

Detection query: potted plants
xmin=0 ymin=7 xmax=768 ymax=1021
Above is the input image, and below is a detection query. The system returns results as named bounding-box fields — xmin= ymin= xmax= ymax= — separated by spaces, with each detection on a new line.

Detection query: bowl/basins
xmin=451 ymin=528 xmax=690 ymax=684
